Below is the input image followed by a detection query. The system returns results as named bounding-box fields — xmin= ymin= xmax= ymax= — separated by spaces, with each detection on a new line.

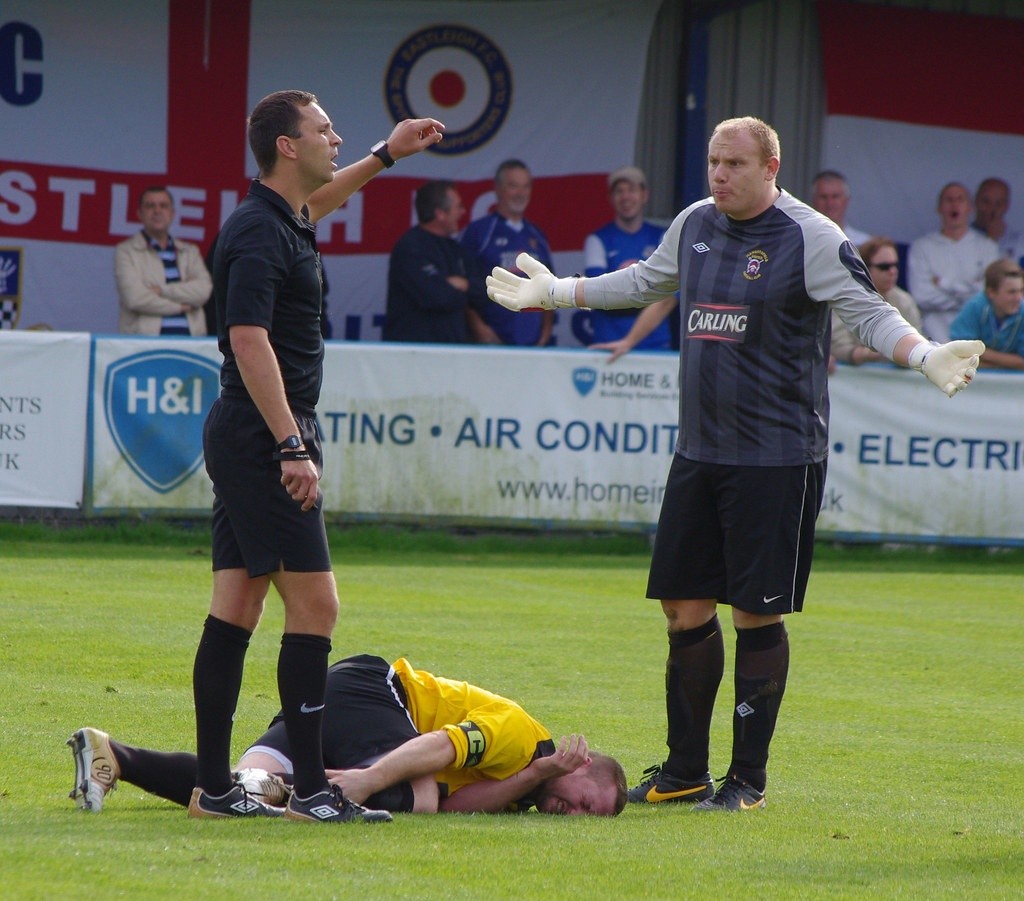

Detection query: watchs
xmin=370 ymin=139 xmax=395 ymax=168
xmin=276 ymin=435 xmax=303 ymax=452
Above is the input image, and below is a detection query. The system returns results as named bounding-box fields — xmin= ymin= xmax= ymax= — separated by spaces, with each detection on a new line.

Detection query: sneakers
xmin=65 ymin=727 xmax=121 ymax=814
xmin=687 ymin=775 xmax=768 ymax=819
xmin=187 ymin=783 xmax=285 ymax=821
xmin=622 ymin=763 xmax=714 ymax=805
xmin=232 ymin=767 xmax=286 ymax=808
xmin=283 ymin=785 xmax=394 ymax=825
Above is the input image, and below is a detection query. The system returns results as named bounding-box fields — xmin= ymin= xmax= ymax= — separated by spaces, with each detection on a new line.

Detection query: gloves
xmin=486 ymin=253 xmax=592 ymax=313
xmin=908 ymin=340 xmax=986 ymax=398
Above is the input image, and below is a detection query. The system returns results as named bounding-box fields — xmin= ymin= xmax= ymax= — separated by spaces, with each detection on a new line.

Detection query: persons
xmin=67 ymin=654 xmax=627 ymax=817
xmin=485 ymin=117 xmax=986 ymax=813
xmin=810 ymin=170 xmax=869 ymax=245
xmin=949 ymin=258 xmax=1024 ymax=370
xmin=457 ymin=158 xmax=553 ymax=346
xmin=114 ymin=186 xmax=213 ymax=336
xmin=187 ymin=90 xmax=445 ymax=824
xmin=831 ymin=236 xmax=924 ymax=364
xmin=382 ymin=181 xmax=490 ymax=345
xmin=584 ymin=166 xmax=672 ymax=351
xmin=588 ymin=291 xmax=680 ymax=364
xmin=906 ymin=181 xmax=998 ymax=344
xmin=971 ymin=177 xmax=1024 ymax=264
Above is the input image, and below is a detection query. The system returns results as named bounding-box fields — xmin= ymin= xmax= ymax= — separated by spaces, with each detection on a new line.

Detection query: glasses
xmin=874 ymin=262 xmax=902 ymax=271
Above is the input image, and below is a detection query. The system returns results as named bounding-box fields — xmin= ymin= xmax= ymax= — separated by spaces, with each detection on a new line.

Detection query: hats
xmin=609 ymin=166 xmax=648 ymax=191
